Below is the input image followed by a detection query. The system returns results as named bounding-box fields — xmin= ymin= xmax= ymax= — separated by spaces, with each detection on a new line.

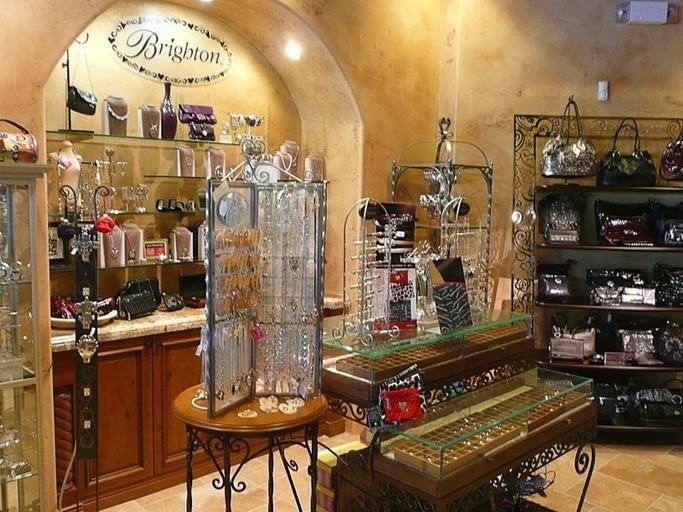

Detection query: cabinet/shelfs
xmin=1 ymin=115 xmax=683 ymax=512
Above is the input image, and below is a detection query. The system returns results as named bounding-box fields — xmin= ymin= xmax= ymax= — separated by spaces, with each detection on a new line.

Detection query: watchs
xmin=231 ymin=115 xmax=261 ymax=130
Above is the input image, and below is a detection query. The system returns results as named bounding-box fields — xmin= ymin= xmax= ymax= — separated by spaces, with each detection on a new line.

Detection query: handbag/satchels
xmin=595 ymin=377 xmax=682 ymax=428
xmin=66 ymin=84 xmax=98 ymax=117
xmin=538 ymin=186 xmax=583 ymax=247
xmin=592 ymin=196 xmax=682 ymax=247
xmin=543 ymin=306 xmax=683 ymax=368
xmin=177 ymin=102 xmax=218 ymax=141
xmin=538 ymin=98 xmax=601 ymax=177
xmin=373 ymin=363 xmax=430 ymax=426
xmin=659 ymin=125 xmax=682 ymax=181
xmin=114 ymin=274 xmax=163 ymax=322
xmin=595 ymin=116 xmax=656 ymax=188
xmin=177 ymin=271 xmax=206 ymax=311
xmin=536 ymin=255 xmax=681 ymax=310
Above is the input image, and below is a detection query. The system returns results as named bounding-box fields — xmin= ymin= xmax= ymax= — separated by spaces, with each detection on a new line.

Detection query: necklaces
xmin=198 ymin=185 xmax=317 ymax=401
xmin=110 ymin=224 xmax=139 ymax=260
xmin=210 ymin=149 xmax=223 ymax=179
xmin=104 ymin=98 xmax=159 ymax=138
xmin=179 ymin=146 xmax=193 ymax=168
xmin=275 ymin=145 xmax=323 ymax=180
xmin=177 ymin=230 xmax=188 ymax=256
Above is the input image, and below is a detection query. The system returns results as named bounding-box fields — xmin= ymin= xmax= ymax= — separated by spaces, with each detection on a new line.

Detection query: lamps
xmin=615 ymin=0 xmax=678 ymax=24
